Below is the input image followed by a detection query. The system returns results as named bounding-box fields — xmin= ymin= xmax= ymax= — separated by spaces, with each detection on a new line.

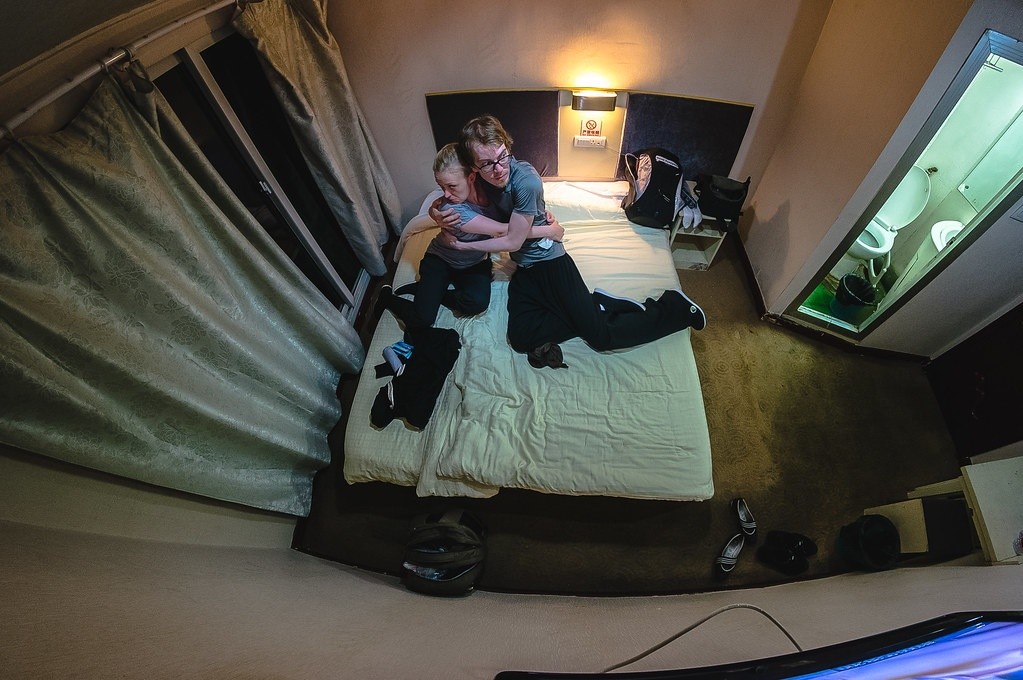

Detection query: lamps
xmin=570 ymin=90 xmax=617 ymax=111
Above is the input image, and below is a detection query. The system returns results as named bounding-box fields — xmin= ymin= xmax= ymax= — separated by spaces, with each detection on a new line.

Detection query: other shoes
xmin=716 ymin=533 xmax=745 ymax=575
xmin=394 ymin=281 xmax=420 ymax=295
xmin=730 ymin=497 xmax=757 ymax=537
xmin=372 ymin=285 xmax=392 ymax=317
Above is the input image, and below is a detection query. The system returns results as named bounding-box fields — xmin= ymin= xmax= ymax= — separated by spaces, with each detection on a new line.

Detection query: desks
xmin=906 ymin=455 xmax=1022 ymax=566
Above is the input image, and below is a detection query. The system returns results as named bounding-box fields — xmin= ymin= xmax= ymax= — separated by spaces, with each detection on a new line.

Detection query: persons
xmin=428 ymin=115 xmax=707 ymax=352
xmin=373 ymin=142 xmax=565 ymax=329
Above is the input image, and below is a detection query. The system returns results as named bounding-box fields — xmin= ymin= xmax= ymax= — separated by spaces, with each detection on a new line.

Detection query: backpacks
xmin=401 ymin=518 xmax=485 ymax=598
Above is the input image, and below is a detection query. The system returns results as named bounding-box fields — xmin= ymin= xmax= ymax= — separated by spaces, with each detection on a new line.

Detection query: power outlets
xmin=574 ymin=136 xmax=606 ymax=148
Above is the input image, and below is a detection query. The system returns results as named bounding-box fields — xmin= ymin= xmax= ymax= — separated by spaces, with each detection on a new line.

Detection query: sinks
xmin=930 ymin=220 xmax=964 ymax=253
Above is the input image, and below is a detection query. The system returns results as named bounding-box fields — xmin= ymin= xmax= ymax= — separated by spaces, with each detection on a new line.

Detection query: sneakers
xmin=593 ymin=288 xmax=646 ymax=314
xmin=668 ymin=289 xmax=706 ymax=331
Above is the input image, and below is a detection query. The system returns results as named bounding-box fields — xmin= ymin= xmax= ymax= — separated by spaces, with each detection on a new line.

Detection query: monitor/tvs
xmin=493 ymin=610 xmax=1022 ymax=680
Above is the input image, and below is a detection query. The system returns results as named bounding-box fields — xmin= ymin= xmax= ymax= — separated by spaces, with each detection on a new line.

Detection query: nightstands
xmin=668 ymin=180 xmax=732 ymax=271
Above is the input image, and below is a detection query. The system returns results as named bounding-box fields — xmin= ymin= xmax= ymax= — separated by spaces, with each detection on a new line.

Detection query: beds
xmin=343 ymin=88 xmax=756 ymax=503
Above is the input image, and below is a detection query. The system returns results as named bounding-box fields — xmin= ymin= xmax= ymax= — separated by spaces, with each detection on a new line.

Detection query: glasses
xmin=474 ymin=149 xmax=513 ymax=173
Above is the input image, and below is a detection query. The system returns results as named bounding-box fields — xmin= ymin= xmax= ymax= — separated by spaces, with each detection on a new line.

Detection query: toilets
xmin=829 ymin=165 xmax=932 ymax=280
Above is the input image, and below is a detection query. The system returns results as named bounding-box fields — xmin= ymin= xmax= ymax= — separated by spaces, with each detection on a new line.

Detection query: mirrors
xmin=957 ymin=109 xmax=1023 ymax=214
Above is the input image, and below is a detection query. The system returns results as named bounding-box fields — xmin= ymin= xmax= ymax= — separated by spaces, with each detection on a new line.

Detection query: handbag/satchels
xmin=624 ymin=147 xmax=681 ymax=229
xmin=697 ymin=174 xmax=751 ymax=218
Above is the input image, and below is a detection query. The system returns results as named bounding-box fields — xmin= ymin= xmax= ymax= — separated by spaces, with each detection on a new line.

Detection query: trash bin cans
xmin=836 ymin=514 xmax=899 ymax=573
xmin=829 ymin=273 xmax=876 ymax=320
